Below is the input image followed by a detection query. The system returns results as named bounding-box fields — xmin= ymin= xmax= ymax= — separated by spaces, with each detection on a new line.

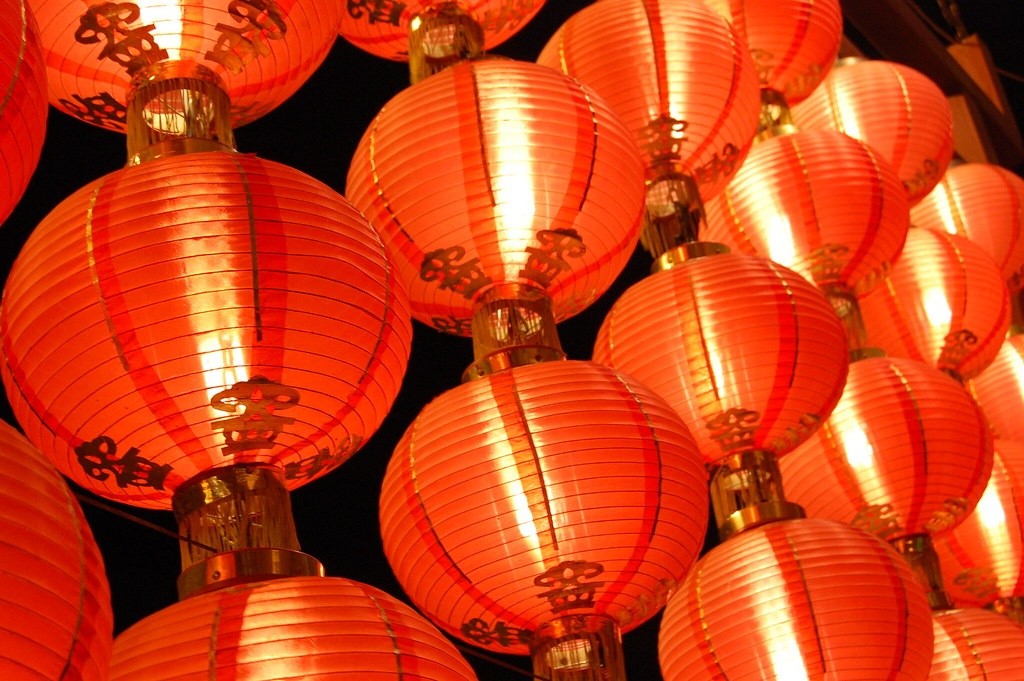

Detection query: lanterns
xmin=0 ymin=0 xmax=1024 ymax=681
xmin=0 ymin=137 xmax=412 ymax=571
